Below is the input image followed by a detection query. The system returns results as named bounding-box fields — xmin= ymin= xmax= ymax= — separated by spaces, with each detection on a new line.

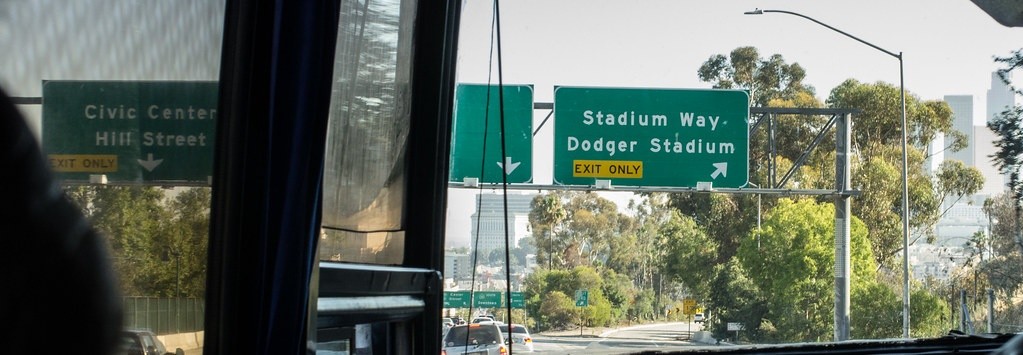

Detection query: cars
xmin=117 ymin=330 xmax=186 ymax=355
xmin=479 ymin=321 xmax=503 ymax=326
xmin=694 ymin=313 xmax=705 ymax=324
xmin=477 ymin=314 xmax=495 ymax=324
xmin=442 ymin=318 xmax=454 ymax=336
xmin=441 ymin=322 xmax=508 ymax=355
xmin=497 ymin=325 xmax=534 ymax=355
xmin=472 ymin=317 xmax=493 ymax=324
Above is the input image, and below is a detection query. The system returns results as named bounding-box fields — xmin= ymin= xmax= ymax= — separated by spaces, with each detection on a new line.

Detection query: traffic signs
xmin=575 ymin=291 xmax=588 ymax=307
xmin=552 ymin=85 xmax=750 ymax=189
xmin=505 ymin=292 xmax=526 ymax=307
xmin=443 ymin=291 xmax=472 ymax=308
xmin=474 ymin=291 xmax=502 ymax=307
xmin=39 ymin=79 xmax=217 ymax=186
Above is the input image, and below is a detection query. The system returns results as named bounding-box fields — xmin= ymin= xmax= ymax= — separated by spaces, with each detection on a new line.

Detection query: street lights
xmin=744 ymin=7 xmax=911 ymax=341
